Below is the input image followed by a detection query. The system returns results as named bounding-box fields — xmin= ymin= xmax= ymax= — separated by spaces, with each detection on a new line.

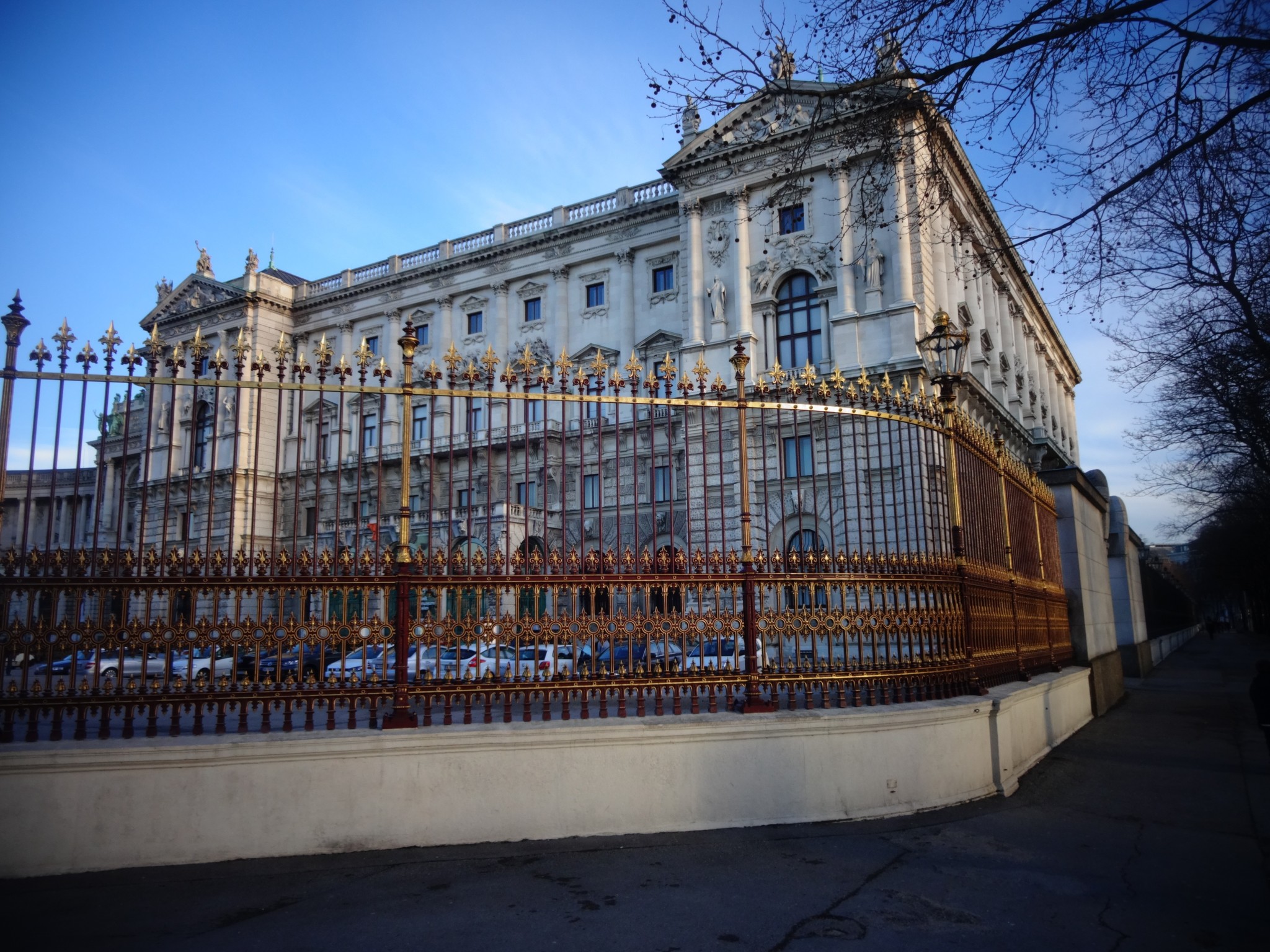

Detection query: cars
xmin=365 ymin=641 xmax=448 ymax=686
xmin=678 ymin=634 xmax=770 ymax=674
xmin=579 ymin=639 xmax=682 ymax=678
xmin=504 ymin=644 xmax=591 ymax=682
xmin=433 ymin=643 xmax=529 ymax=682
xmin=34 ymin=642 xmax=395 ymax=682
